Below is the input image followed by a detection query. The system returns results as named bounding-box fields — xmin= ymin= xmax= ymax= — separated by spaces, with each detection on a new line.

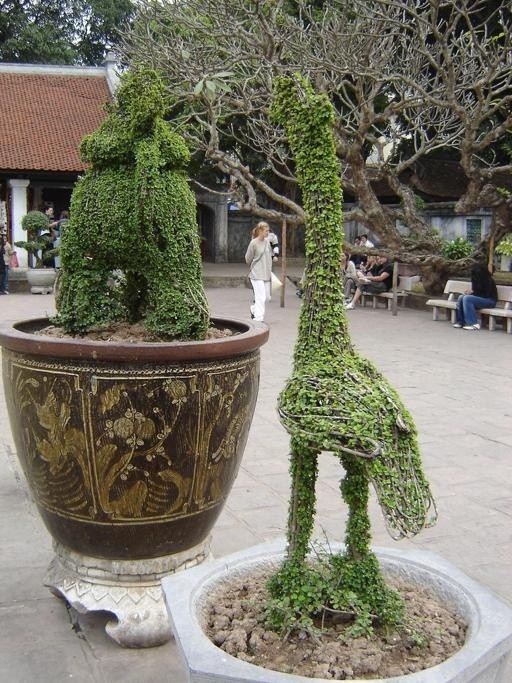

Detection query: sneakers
xmin=463 ymin=323 xmax=480 ymax=330
xmin=343 ymin=303 xmax=355 ymax=310
xmin=452 ymin=322 xmax=467 ymax=328
xmin=358 ymin=278 xmax=372 ymax=285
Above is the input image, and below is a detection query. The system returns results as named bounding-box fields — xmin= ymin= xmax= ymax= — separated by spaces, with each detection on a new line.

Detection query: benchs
xmin=426 ymin=280 xmax=511 ymax=334
xmin=352 ymin=275 xmax=420 ymax=309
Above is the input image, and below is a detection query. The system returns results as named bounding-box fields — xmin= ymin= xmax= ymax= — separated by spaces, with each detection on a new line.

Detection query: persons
xmin=341 ymin=233 xmax=400 ymax=309
xmin=56 ymin=208 xmax=70 ymax=236
xmin=451 ymin=249 xmax=497 ymax=331
xmin=251 ymin=227 xmax=280 ymax=302
xmin=244 ymin=220 xmax=274 ymax=322
xmin=36 ymin=204 xmax=60 ymax=240
xmin=0 ymin=233 xmax=17 ymax=295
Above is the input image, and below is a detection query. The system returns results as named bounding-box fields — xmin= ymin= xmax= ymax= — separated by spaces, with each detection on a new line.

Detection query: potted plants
xmin=2 ymin=68 xmax=271 ymax=648
xmin=161 ymin=69 xmax=510 ymax=683
xmin=13 ymin=208 xmax=60 ymax=295
